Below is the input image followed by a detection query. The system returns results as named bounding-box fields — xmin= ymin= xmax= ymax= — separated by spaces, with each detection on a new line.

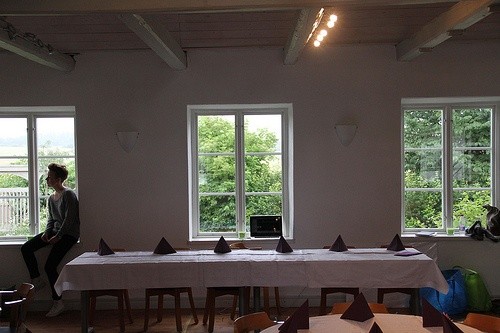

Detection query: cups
xmin=245 ymin=232 xmax=252 ymax=239
xmin=447 ymin=228 xmax=453 ymax=234
xmin=239 ymin=232 xmax=245 ymax=238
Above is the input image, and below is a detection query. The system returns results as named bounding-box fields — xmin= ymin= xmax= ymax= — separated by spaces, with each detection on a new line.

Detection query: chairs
xmin=0 ymin=283 xmax=35 ymax=333
xmin=144 ymin=248 xmax=199 ymax=333
xmin=378 ymin=245 xmax=419 ymax=312
xmin=228 ymin=241 xmax=281 ymax=319
xmin=233 ymin=310 xmax=278 ymax=333
xmin=462 ymin=313 xmax=500 ymax=333
xmin=321 ymin=246 xmax=359 ymax=314
xmin=87 ymin=249 xmax=134 ymax=333
xmin=331 ymin=303 xmax=388 ymax=315
xmin=202 ymin=247 xmax=247 ymax=333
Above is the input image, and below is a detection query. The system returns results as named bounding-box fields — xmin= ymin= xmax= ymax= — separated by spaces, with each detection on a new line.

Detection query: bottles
xmin=459 ymin=215 xmax=465 ymax=235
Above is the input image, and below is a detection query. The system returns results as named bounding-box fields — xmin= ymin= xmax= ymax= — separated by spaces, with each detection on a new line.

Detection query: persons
xmin=21 ymin=163 xmax=79 ymax=319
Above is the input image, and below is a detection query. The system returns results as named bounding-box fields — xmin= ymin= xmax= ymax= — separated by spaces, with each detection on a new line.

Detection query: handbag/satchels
xmin=482 ymin=204 xmax=500 ymax=242
xmin=420 ymin=269 xmax=470 ymax=317
xmin=452 ymin=265 xmax=492 ymax=311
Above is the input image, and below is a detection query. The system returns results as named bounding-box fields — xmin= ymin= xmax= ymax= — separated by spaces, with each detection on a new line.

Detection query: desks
xmin=54 ymin=247 xmax=449 ymax=333
xmin=259 ymin=313 xmax=484 ymax=333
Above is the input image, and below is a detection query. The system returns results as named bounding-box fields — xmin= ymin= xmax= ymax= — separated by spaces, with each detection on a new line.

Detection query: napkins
xmin=329 ymin=234 xmax=348 ymax=253
xmin=96 ymin=235 xmax=293 ymax=255
xmin=368 ymin=321 xmax=383 ymax=333
xmin=422 ymin=296 xmax=442 ymax=327
xmin=279 ymin=298 xmax=310 ymax=333
xmin=442 ymin=314 xmax=463 ymax=333
xmin=387 ymin=232 xmax=406 ymax=252
xmin=340 ymin=292 xmax=374 ymax=321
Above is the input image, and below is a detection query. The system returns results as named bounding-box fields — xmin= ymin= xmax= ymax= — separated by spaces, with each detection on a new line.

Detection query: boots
xmin=46 ymin=300 xmax=64 ymax=318
xmin=31 ymin=276 xmax=44 ymax=288
